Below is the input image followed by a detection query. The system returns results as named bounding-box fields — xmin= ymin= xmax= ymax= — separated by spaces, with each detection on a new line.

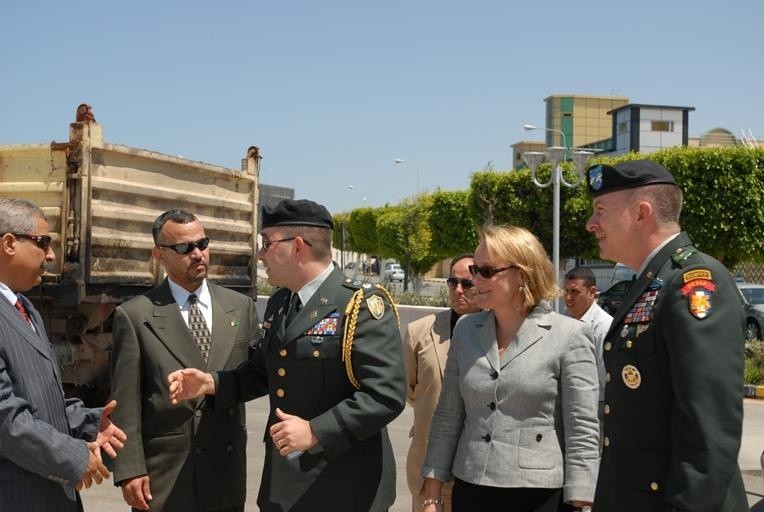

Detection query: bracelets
xmin=421 ymin=499 xmax=444 ymax=508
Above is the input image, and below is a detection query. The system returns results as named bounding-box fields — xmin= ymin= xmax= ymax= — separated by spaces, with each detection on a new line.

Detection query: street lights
xmin=520 ymin=124 xmax=605 ymax=313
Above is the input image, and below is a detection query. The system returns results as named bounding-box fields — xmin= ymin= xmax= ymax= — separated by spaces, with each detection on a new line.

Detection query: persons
xmin=168 ymin=198 xmax=407 ymax=511
xmin=0 ymin=199 xmax=127 ymax=512
xmin=559 ymin=265 xmax=614 ymax=401
xmin=105 ymin=208 xmax=264 ymax=512
xmin=420 ymin=225 xmax=602 ymax=512
xmin=404 ymin=254 xmax=482 ymax=512
xmin=590 ymin=157 xmax=748 ymax=511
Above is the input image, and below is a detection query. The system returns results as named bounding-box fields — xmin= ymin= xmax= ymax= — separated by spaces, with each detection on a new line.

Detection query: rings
xmin=276 ymin=440 xmax=285 ymax=445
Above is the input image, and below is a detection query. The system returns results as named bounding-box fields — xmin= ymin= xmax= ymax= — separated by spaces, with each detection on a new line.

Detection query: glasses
xmin=262 ymin=238 xmax=312 ymax=251
xmin=0 ymin=233 xmax=51 ymax=250
xmin=159 ymin=236 xmax=209 ymax=254
xmin=468 ymin=265 xmax=513 ymax=278
xmin=447 ymin=278 xmax=474 ymax=289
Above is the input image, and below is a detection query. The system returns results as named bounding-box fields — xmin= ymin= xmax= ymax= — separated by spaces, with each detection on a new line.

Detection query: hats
xmin=262 ymin=199 xmax=334 ymax=228
xmin=586 ymin=158 xmax=677 ymax=197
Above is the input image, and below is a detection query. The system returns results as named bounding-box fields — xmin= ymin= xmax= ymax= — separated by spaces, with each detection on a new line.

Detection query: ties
xmin=188 ymin=294 xmax=211 ymax=365
xmin=285 ymin=293 xmax=301 ymax=328
xmin=625 ymin=273 xmax=637 ymax=288
xmin=15 ymin=300 xmax=31 ymax=325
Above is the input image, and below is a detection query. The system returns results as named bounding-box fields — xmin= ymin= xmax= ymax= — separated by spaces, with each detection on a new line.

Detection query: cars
xmin=735 ymin=282 xmax=764 ymax=342
xmin=597 ymin=280 xmax=632 ymax=317
xmin=384 ymin=264 xmax=410 ymax=282
